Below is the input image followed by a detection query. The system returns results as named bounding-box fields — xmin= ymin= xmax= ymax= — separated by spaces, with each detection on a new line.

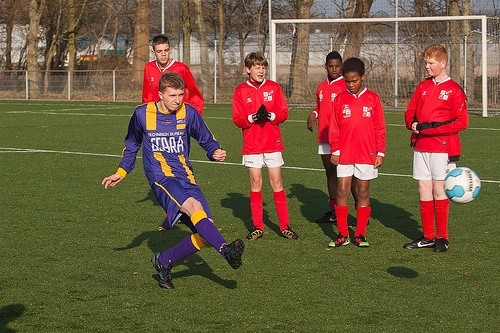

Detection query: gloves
xmin=252 ymin=103 xmax=272 ymax=122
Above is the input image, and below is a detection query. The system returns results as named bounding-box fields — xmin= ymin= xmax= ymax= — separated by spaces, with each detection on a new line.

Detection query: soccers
xmin=444 ymin=167 xmax=482 ymax=204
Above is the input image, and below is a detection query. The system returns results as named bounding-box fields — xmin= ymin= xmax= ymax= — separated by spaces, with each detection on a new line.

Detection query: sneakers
xmin=279 ymin=226 xmax=299 ymax=239
xmin=150 ymin=250 xmax=176 ymax=290
xmin=245 ymin=227 xmax=264 ymax=240
xmin=329 ymin=232 xmax=350 ymax=247
xmin=352 ymin=234 xmax=370 ymax=247
xmin=224 ymin=238 xmax=244 ymax=269
xmin=403 ymin=235 xmax=436 ymax=249
xmin=159 ymin=218 xmax=172 ymax=231
xmin=315 ymin=210 xmax=338 ymax=224
xmin=433 ymin=238 xmax=449 ymax=253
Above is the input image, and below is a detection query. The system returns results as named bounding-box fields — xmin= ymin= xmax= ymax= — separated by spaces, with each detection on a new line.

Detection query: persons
xmin=404 ymin=44 xmax=469 ymax=252
xmin=307 ymin=50 xmax=347 ymax=224
xmin=328 ymin=56 xmax=386 ymax=247
xmin=142 ymin=36 xmax=204 ymax=230
xmin=105 ymin=76 xmax=244 ymax=289
xmin=232 ymin=51 xmax=300 ymax=241
